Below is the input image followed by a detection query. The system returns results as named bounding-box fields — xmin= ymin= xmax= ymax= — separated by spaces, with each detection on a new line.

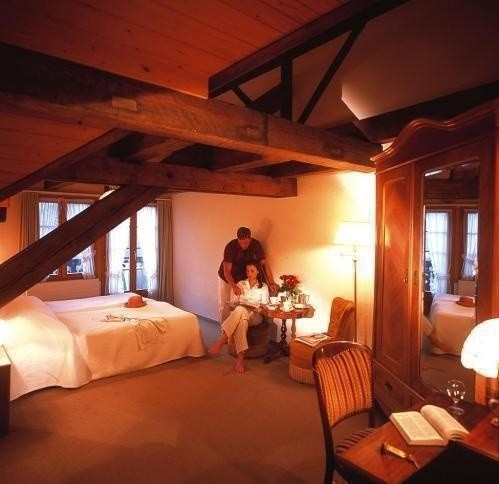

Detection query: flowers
xmin=278 ymin=274 xmax=300 ymax=296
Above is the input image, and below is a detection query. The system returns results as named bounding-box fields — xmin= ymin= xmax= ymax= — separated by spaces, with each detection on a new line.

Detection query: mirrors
xmin=416 ymin=137 xmax=482 ymax=404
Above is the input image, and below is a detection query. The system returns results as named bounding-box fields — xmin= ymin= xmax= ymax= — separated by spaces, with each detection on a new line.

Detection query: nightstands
xmin=0 ymin=344 xmax=12 ymax=436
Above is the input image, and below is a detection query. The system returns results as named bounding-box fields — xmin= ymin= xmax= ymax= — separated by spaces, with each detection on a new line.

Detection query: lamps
xmin=331 ymin=219 xmax=376 ymax=342
xmin=461 ymin=318 xmax=499 ymax=427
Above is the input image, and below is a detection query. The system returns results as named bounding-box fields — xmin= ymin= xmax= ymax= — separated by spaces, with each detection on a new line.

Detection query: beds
xmin=0 ymin=292 xmax=207 ymax=402
xmin=429 ymin=292 xmax=476 ymax=356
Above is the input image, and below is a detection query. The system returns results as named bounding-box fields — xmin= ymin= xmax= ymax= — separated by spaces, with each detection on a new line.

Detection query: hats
xmin=457 ymin=297 xmax=475 ymax=307
xmin=125 ymin=296 xmax=146 ymax=308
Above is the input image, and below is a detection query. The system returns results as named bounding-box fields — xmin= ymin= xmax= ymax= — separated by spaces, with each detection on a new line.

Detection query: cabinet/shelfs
xmin=369 ymin=99 xmax=499 ymax=418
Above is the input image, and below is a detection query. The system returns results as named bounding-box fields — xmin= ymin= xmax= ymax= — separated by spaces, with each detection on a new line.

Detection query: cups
xmin=267 ymin=296 xmax=280 ymax=303
xmin=281 ymin=296 xmax=288 ymax=302
xmin=283 ymin=302 xmax=292 ymax=310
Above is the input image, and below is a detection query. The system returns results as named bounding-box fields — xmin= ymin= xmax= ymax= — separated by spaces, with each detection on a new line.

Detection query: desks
xmin=259 ymin=306 xmax=314 ymax=363
xmin=338 ymin=393 xmax=498 ymax=484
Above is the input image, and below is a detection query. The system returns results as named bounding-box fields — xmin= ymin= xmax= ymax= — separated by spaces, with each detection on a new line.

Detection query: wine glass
xmin=447 ymin=379 xmax=466 ymax=417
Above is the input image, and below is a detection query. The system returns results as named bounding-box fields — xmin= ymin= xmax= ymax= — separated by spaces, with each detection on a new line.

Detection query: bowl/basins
xmin=267 ymin=305 xmax=278 ymax=311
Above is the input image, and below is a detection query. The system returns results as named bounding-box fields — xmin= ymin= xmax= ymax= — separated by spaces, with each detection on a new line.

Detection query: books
xmin=295 ymin=331 xmax=331 ymax=347
xmin=388 ymin=403 xmax=469 ymax=447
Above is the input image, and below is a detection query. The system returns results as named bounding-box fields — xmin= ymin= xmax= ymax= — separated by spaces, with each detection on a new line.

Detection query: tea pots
xmin=298 ymin=294 xmax=309 ymax=305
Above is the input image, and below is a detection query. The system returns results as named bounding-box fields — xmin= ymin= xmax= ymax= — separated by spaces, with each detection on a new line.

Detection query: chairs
xmin=227 ymin=283 xmax=280 ymax=360
xmin=290 ymin=297 xmax=355 ymax=384
xmin=311 ymin=341 xmax=379 ymax=484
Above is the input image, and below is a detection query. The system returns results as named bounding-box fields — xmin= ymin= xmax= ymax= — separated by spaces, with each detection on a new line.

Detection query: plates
xmin=269 ymin=303 xmax=280 ymax=305
xmin=280 ymin=307 xmax=293 ymax=312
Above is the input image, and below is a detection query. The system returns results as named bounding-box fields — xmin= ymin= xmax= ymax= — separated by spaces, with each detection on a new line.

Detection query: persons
xmin=421 ymin=315 xmax=452 ymax=356
xmin=208 ymin=259 xmax=271 ymax=374
xmin=218 ymin=226 xmax=280 ymax=334
